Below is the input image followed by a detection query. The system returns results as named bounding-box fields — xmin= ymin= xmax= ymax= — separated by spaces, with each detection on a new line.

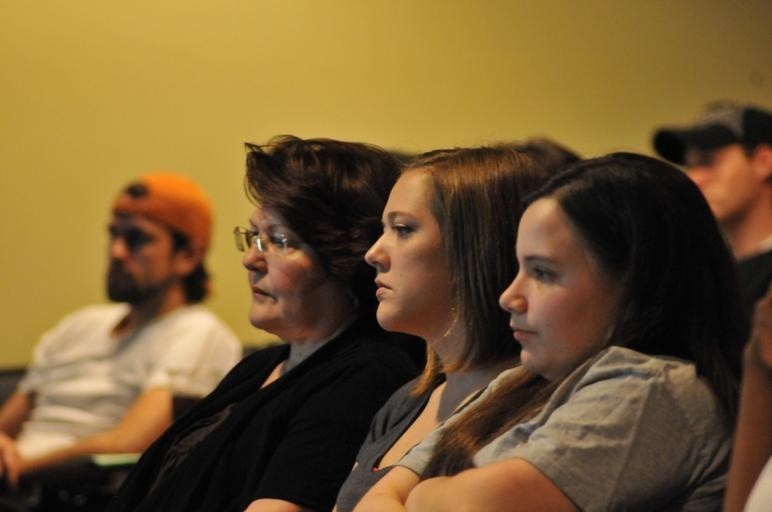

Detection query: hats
xmin=652 ymin=102 xmax=772 ymax=166
xmin=112 ymin=170 xmax=210 ymax=247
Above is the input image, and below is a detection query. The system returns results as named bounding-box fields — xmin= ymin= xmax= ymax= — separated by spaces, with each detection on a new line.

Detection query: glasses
xmin=232 ymin=226 xmax=299 ymax=253
